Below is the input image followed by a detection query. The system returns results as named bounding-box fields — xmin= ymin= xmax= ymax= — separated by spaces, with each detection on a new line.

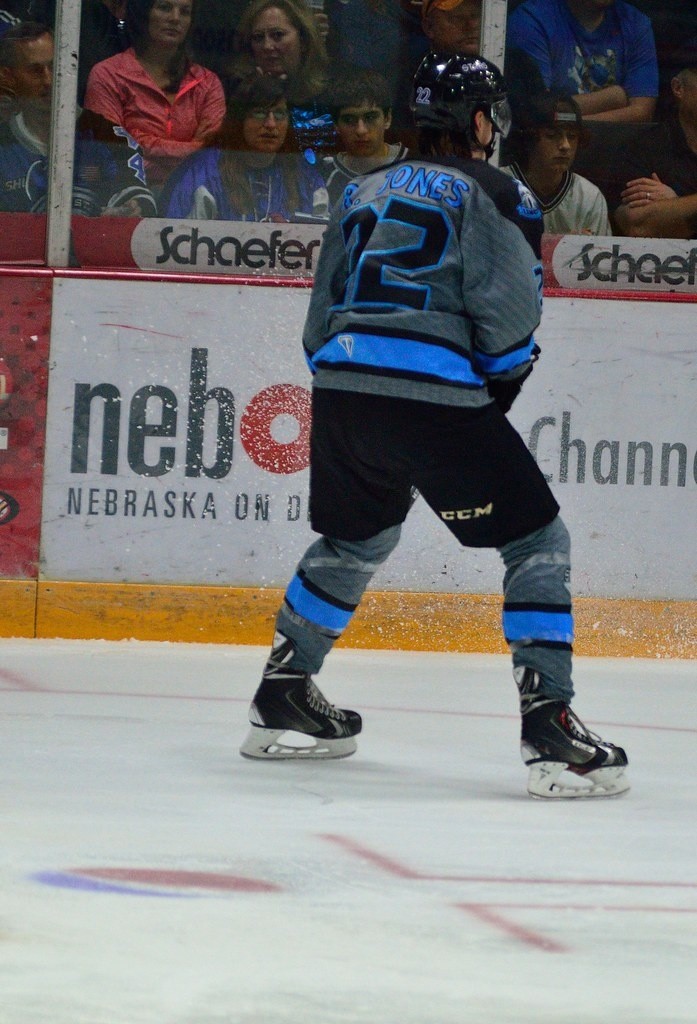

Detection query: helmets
xmin=409 ymin=49 xmax=506 ymax=134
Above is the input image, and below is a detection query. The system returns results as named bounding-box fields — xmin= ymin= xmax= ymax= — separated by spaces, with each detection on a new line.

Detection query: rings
xmin=647 ymin=192 xmax=651 ymax=200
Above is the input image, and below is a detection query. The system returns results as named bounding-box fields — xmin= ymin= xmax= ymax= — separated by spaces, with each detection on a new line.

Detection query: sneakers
xmin=512 ymin=666 xmax=634 ymax=798
xmin=240 ymin=629 xmax=362 ymax=759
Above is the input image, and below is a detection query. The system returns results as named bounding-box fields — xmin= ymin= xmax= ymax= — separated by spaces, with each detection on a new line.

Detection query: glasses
xmin=250 ymin=109 xmax=287 ymax=119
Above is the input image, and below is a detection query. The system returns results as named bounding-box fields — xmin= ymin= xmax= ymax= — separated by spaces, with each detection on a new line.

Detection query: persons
xmin=0 ymin=0 xmax=697 ymax=240
xmin=250 ymin=50 xmax=628 ymax=779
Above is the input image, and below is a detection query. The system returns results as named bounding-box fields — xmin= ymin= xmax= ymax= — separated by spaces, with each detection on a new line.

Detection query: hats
xmin=421 ymin=0 xmax=464 ymax=20
xmin=521 ymin=89 xmax=582 ymax=130
xmin=668 ymin=46 xmax=696 ymax=69
